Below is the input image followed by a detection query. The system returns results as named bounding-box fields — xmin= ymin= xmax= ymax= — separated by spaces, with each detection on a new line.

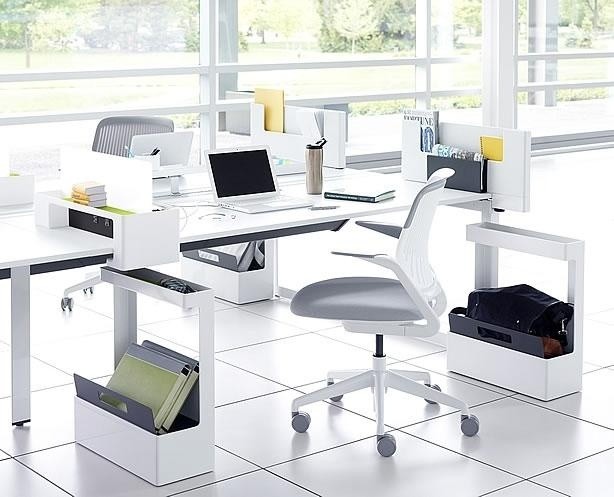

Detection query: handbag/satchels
xmin=466 ymin=284 xmax=574 ymax=358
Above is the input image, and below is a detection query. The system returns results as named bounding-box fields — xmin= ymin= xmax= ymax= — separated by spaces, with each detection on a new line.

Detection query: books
xmin=98 ymin=339 xmax=200 ymax=436
xmin=403 ymin=107 xmax=441 ymax=150
xmin=70 ymin=180 xmax=108 ymax=208
xmin=323 ymin=185 xmax=396 ymax=203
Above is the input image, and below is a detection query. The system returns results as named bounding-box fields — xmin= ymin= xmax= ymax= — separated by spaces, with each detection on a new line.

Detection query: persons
xmin=565 ymin=24 xmax=578 ymax=47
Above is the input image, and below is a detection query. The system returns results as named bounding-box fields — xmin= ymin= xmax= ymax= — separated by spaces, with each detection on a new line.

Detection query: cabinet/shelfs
xmin=447 ymin=222 xmax=584 ymax=402
xmin=75 ymin=265 xmax=215 ymax=487
xmin=400 ymin=120 xmax=532 ymax=214
xmin=224 ymin=88 xmax=347 ymax=169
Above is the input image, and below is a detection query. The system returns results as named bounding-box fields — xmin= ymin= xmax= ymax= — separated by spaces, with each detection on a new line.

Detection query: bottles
xmin=304 ymin=137 xmax=327 ymax=195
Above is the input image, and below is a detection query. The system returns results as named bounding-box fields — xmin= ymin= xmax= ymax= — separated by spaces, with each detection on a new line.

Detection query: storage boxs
xmin=180 ymin=238 xmax=273 ymax=305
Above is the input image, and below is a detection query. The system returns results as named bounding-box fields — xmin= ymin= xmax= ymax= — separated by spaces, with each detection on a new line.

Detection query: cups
xmin=133 ymin=153 xmax=160 ymax=171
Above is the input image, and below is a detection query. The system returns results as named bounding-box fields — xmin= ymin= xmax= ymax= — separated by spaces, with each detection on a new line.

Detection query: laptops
xmin=203 ymin=145 xmax=314 ymax=214
xmin=128 ymin=131 xmax=193 ymax=167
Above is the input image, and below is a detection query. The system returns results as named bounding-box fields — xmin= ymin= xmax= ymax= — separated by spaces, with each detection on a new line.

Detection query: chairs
xmin=61 ymin=116 xmax=174 ymax=311
xmin=290 ymin=167 xmax=480 ymax=457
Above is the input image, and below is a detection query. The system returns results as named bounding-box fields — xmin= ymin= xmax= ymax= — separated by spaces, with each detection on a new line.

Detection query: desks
xmin=0 ymin=168 xmax=497 ymax=425
